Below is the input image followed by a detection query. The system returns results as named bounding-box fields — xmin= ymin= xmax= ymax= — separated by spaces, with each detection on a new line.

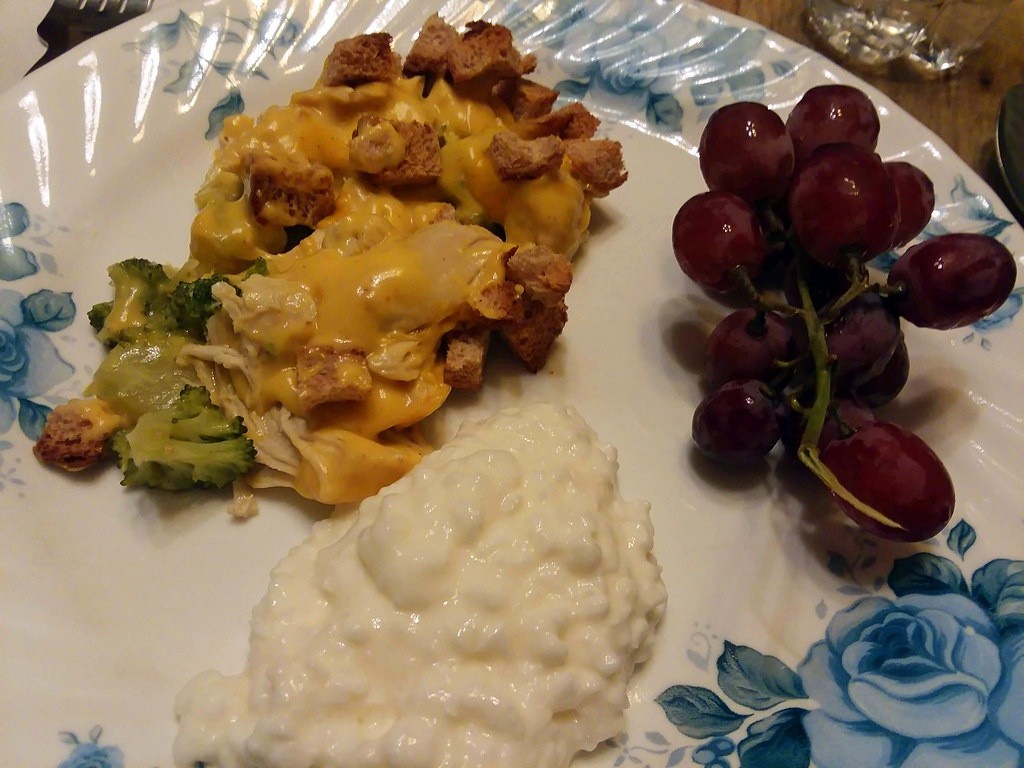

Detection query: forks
xmin=23 ymin=0 xmax=155 ymax=78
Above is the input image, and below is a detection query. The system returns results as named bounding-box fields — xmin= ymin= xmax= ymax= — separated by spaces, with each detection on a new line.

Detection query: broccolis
xmin=87 ymin=258 xmax=270 ymax=491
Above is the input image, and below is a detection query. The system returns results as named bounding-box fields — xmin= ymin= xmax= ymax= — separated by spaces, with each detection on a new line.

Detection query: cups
xmin=804 ymin=0 xmax=945 ymax=71
xmin=873 ymin=0 xmax=1014 ymax=76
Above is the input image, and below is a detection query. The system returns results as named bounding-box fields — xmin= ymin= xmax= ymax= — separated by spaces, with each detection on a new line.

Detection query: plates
xmin=0 ymin=0 xmax=1024 ymax=768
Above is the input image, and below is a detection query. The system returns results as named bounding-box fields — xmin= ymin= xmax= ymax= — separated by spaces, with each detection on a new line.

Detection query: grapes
xmin=672 ymin=84 xmax=1016 ymax=539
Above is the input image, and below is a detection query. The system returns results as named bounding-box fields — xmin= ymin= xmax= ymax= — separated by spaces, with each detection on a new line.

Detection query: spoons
xmin=995 ymin=82 xmax=1024 ymax=222
